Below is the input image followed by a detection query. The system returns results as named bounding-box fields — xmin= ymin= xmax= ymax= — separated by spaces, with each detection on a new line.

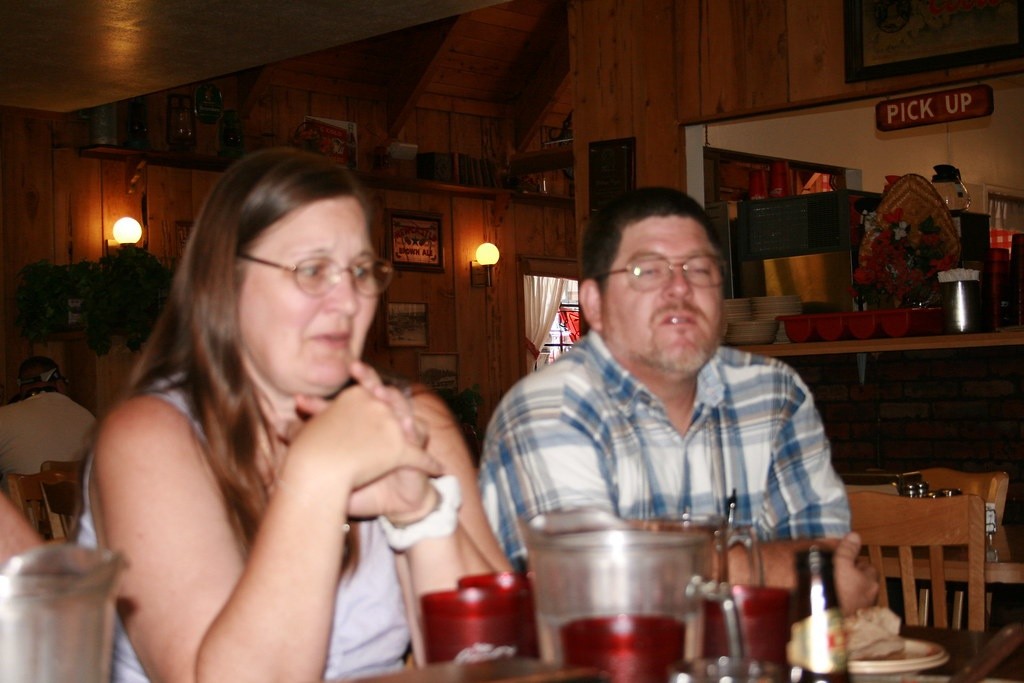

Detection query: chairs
xmin=845 ymin=466 xmax=1010 ymax=633
xmin=0 ymin=459 xmax=87 ymax=542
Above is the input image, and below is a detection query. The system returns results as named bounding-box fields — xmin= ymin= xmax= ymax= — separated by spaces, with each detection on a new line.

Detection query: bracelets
xmin=377 ymin=473 xmax=461 ymax=548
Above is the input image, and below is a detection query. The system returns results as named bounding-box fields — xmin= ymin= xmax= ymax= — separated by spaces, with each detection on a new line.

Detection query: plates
xmin=718 ymin=300 xmax=751 ymax=345
xmin=725 ymin=323 xmax=780 ymax=345
xmin=752 ymin=296 xmax=801 ymax=342
xmin=848 ymin=638 xmax=949 ymax=677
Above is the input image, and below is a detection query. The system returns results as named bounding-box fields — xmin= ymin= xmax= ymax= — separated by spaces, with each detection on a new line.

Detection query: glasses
xmin=234 ymin=254 xmax=394 ymax=296
xmin=593 ymin=258 xmax=728 ymax=292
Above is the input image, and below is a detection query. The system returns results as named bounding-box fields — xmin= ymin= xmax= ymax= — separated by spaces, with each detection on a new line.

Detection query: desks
xmin=855 ymin=526 xmax=1024 ymax=584
xmin=900 ymin=625 xmax=1024 ymax=683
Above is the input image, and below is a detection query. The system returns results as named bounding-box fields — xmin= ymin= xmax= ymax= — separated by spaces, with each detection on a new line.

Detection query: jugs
xmin=932 ymin=164 xmax=970 ymax=217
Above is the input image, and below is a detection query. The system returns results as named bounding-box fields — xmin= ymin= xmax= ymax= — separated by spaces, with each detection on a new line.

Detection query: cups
xmin=0 ymin=544 xmax=119 ymax=683
xmin=528 ymin=508 xmax=705 ymax=683
xmin=458 ymin=572 xmax=536 ymax=655
xmin=940 ymin=281 xmax=983 ymax=334
xmin=710 ymin=585 xmax=789 ymax=683
xmin=422 ymin=591 xmax=527 ymax=667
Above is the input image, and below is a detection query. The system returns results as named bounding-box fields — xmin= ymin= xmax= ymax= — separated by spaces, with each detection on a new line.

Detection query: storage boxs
xmin=416 ymin=152 xmax=452 ymax=182
xmin=736 ymin=191 xmax=848 ymax=260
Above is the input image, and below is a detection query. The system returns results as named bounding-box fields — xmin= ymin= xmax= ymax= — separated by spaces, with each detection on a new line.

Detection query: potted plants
xmin=15 ymin=243 xmax=173 ymax=358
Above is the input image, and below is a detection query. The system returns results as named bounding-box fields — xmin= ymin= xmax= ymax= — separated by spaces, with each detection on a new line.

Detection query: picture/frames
xmin=415 ymin=351 xmax=460 ymax=392
xmin=385 ymin=207 xmax=446 ymax=273
xmin=385 ymin=302 xmax=430 ymax=349
xmin=842 ymin=0 xmax=1024 ymax=84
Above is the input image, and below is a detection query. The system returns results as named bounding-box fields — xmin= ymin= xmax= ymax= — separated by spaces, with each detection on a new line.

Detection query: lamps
xmin=124 ymin=97 xmax=152 ymax=150
xmin=386 ymin=142 xmax=419 ymax=160
xmin=106 ymin=216 xmax=142 ymax=258
xmin=470 ymin=241 xmax=500 ymax=286
xmin=167 ymin=93 xmax=197 ymax=152
xmin=218 ymin=107 xmax=244 ymax=158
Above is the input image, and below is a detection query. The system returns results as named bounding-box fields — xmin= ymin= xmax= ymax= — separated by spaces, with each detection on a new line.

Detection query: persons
xmin=0 ymin=356 xmax=98 ymax=535
xmin=477 ymin=186 xmax=879 ymax=613
xmin=81 ymin=151 xmax=513 ymax=683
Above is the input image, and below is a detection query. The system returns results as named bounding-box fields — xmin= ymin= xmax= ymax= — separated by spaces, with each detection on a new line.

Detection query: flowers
xmin=287 ymin=125 xmax=333 ymax=156
xmin=848 ymin=175 xmax=954 ymax=310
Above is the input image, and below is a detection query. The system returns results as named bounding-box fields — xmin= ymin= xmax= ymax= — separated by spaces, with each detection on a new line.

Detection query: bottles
xmin=785 ymin=551 xmax=850 ymax=683
xmin=903 ymin=484 xmax=960 ymax=498
